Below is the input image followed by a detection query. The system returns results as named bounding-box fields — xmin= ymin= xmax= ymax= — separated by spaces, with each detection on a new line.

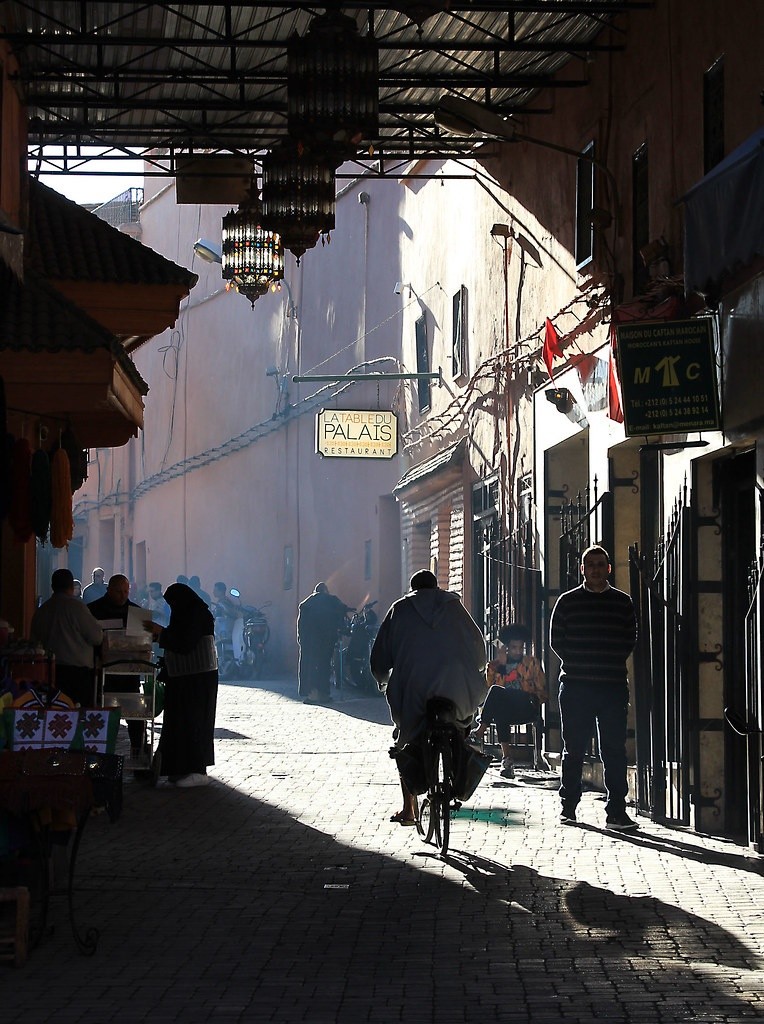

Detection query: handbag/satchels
xmin=86 ymin=750 xmax=125 ymax=824
xmin=388 ymin=739 xmax=429 ymax=796
xmin=8 ymin=647 xmax=48 ymax=686
xmin=11 ymin=749 xmax=94 ymax=814
xmin=451 ymin=732 xmax=494 ymax=802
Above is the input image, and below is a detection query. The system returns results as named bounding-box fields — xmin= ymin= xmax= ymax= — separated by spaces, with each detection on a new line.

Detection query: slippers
xmin=390 ymin=811 xmax=414 ymax=822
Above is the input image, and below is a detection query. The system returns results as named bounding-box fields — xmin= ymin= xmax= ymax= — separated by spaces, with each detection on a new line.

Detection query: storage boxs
xmin=100 ymin=692 xmax=152 ymax=717
xmin=100 ymin=629 xmax=153 ymax=672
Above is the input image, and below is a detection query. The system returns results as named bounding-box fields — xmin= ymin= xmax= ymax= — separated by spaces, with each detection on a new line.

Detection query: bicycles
xmin=399 ymin=698 xmax=462 ymax=856
xmin=328 ymin=608 xmax=358 ymax=688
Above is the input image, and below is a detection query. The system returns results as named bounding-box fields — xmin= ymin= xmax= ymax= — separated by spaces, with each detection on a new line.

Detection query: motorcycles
xmin=211 ymin=588 xmax=272 ymax=679
xmin=342 ymin=600 xmax=383 ymax=693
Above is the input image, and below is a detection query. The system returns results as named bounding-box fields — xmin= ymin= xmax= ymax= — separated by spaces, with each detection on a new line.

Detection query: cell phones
xmin=495 ymin=665 xmax=506 ymax=674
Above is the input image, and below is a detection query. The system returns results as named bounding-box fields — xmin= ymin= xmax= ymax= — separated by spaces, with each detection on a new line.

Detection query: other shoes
xmin=303 ymin=698 xmax=319 ymax=704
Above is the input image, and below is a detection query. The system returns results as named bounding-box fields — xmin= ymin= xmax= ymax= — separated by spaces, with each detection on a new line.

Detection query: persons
xmin=470 ymin=625 xmax=545 ymax=779
xmin=369 ymin=570 xmax=487 ymax=823
xmin=144 ymin=575 xmax=238 ymax=663
xmin=550 ymin=545 xmax=640 ymax=831
xmin=37 ymin=567 xmax=147 ymax=762
xmin=296 ymin=583 xmax=349 ymax=705
xmin=142 ymin=583 xmax=219 ymax=787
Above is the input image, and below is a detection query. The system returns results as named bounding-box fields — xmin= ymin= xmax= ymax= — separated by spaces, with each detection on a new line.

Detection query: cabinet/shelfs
xmin=94 ymin=656 xmax=157 ymax=770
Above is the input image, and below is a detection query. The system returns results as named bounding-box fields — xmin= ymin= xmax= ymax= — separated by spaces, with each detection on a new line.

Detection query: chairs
xmin=481 ymin=705 xmax=541 ymax=771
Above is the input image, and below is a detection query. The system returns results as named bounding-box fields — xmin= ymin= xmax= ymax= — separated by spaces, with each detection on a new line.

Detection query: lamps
xmin=222 ymin=8 xmax=380 ymax=310
xmin=195 ymin=239 xmax=297 ymax=318
xmin=434 ymin=95 xmax=622 ymax=238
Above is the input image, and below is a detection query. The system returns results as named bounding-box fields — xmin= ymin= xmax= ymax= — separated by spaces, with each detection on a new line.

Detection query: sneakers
xmin=559 ymin=809 xmax=576 ymax=823
xmin=465 ymin=730 xmax=482 ymax=746
xmin=175 ymin=773 xmax=210 ymax=787
xmin=606 ymin=812 xmax=639 ymax=830
xmin=499 ymin=757 xmax=515 ymax=779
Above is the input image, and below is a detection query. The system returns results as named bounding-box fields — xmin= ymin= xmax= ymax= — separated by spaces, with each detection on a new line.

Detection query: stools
xmin=1 ymin=886 xmax=30 ymax=968
